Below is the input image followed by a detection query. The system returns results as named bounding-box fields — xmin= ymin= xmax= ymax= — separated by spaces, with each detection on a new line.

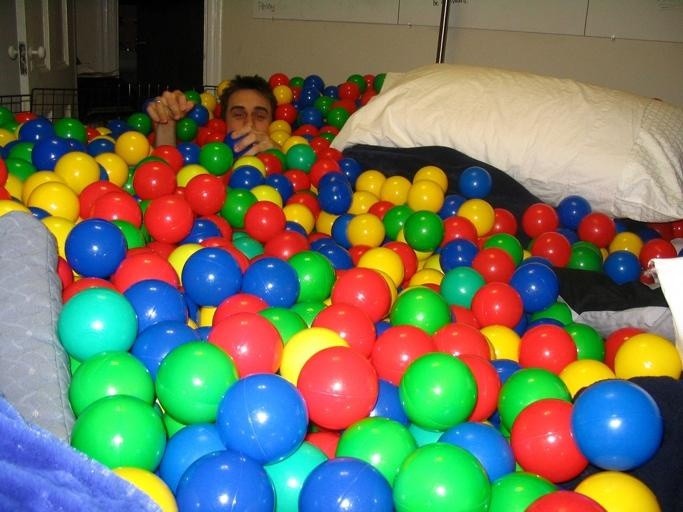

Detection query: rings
xmin=156 ymin=100 xmax=161 ymax=102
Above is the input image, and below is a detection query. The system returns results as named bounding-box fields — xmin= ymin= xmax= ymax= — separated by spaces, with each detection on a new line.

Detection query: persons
xmin=146 ymin=74 xmax=282 ymax=155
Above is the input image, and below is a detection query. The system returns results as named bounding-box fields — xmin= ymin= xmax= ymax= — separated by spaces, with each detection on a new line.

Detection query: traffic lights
xmin=327 ymin=62 xmax=682 ymax=225
